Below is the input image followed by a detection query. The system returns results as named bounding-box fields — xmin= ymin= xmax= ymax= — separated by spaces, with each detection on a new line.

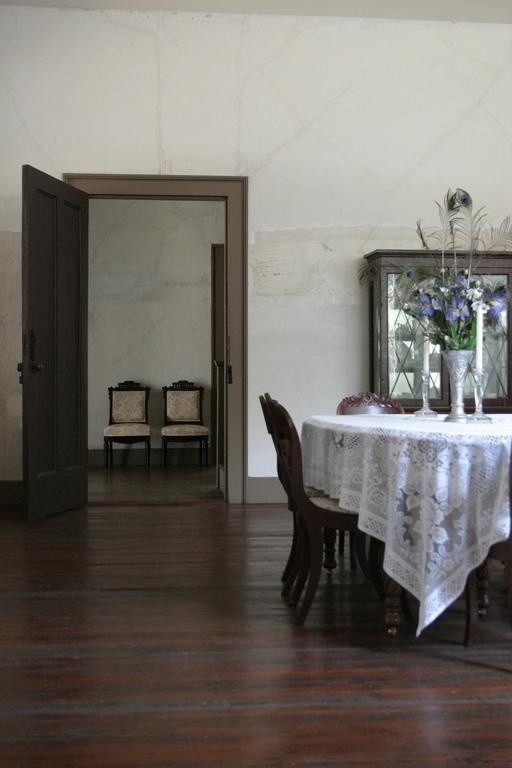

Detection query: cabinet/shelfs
xmin=363 ymin=244 xmax=512 ymax=413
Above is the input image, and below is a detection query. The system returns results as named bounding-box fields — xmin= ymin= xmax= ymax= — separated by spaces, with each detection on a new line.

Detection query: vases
xmin=440 ymin=350 xmax=475 ymax=422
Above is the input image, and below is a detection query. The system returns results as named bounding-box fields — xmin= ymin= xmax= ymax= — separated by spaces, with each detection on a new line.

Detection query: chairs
xmin=335 ymin=390 xmax=404 ymax=415
xmin=103 ymin=380 xmax=152 ymax=482
xmin=259 ymin=392 xmax=385 ymax=621
xmin=160 ymin=380 xmax=211 ymax=483
xmin=464 ymin=541 xmax=511 ymax=648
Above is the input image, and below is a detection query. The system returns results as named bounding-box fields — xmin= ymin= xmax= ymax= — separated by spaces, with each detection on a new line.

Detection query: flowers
xmin=358 ymin=186 xmax=512 ymax=350
